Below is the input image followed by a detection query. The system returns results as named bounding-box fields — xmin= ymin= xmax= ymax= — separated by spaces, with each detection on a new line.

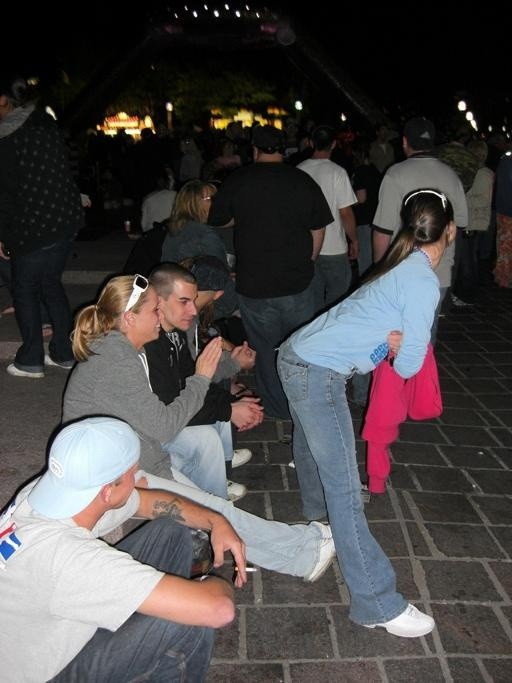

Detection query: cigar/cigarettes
xmin=235 ymin=567 xmax=257 ymax=572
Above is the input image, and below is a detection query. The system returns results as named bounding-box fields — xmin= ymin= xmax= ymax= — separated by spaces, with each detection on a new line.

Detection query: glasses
xmin=201 ymin=194 xmax=217 ymax=204
xmin=1 ymin=246 xmax=15 ymax=256
xmin=125 ymin=274 xmax=149 ymax=313
xmin=254 ymin=144 xmax=286 ymax=155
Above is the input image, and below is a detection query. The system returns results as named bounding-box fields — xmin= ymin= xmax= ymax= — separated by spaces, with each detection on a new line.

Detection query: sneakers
xmin=44 ymin=354 xmax=73 ymax=369
xmin=227 ymin=481 xmax=248 ymax=502
xmin=362 ymin=603 xmax=435 ymax=638
xmin=308 ymin=521 xmax=336 ymax=583
xmin=232 ymin=448 xmax=252 ymax=468
xmin=8 ymin=364 xmax=45 ymax=378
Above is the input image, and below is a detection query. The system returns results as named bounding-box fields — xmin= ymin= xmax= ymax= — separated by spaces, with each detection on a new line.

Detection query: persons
xmin=2 ymin=87 xmax=511 ymax=420
xmin=63 ymin=272 xmax=337 ymax=584
xmin=277 ymin=189 xmax=456 ymax=637
xmin=1 ymin=415 xmax=246 ymax=683
xmin=142 ymin=260 xmax=263 ymax=470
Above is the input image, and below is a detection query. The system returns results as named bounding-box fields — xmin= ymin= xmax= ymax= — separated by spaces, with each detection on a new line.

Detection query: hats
xmin=191 ymin=256 xmax=229 ymax=291
xmin=404 ymin=116 xmax=438 ymax=151
xmin=27 ymin=417 xmax=141 ymax=520
